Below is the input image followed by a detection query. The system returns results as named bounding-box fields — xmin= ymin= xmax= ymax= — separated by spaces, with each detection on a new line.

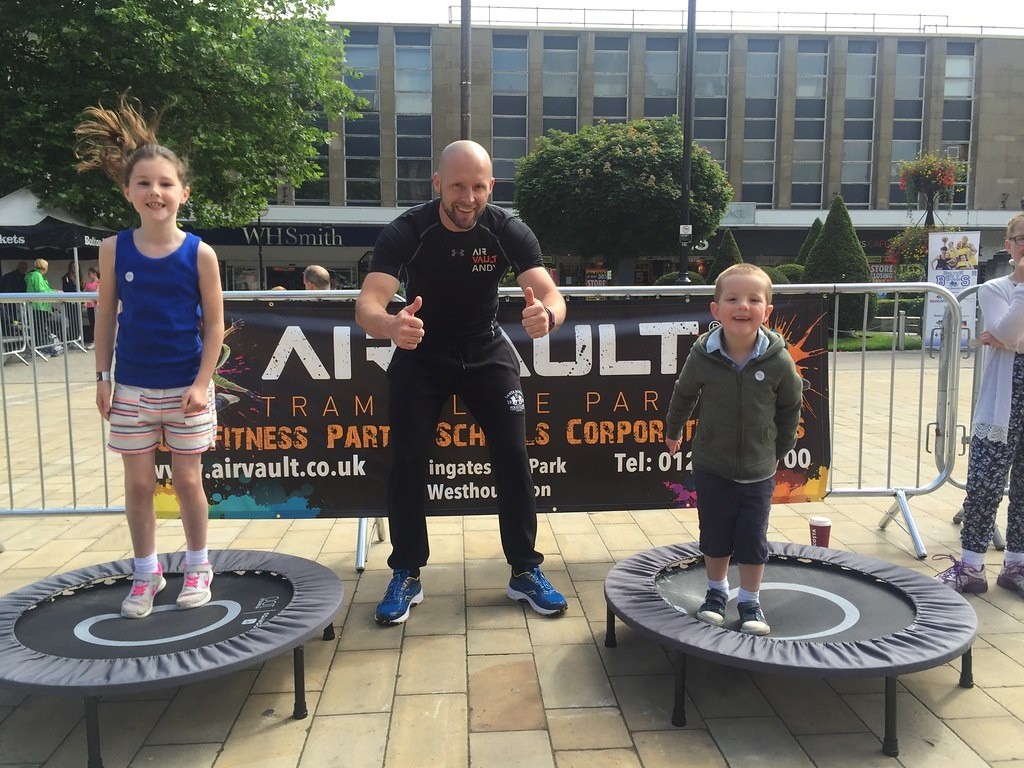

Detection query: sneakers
xmin=996 ymin=560 xmax=1024 ymax=599
xmin=737 ymin=601 xmax=771 ymax=636
xmin=176 ymin=561 xmax=213 ymax=609
xmin=121 ymin=562 xmax=167 ymax=619
xmin=507 ymin=568 xmax=568 ymax=616
xmin=697 ymin=587 xmax=729 ymax=628
xmin=930 ymin=554 xmax=988 ymax=595
xmin=375 ymin=568 xmax=424 ymax=625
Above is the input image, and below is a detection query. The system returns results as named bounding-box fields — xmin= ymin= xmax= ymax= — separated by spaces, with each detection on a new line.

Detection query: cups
xmin=809 ymin=515 xmax=832 ymax=548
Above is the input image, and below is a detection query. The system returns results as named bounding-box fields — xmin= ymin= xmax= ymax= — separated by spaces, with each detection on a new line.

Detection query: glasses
xmin=1009 ymin=236 xmax=1024 ymax=246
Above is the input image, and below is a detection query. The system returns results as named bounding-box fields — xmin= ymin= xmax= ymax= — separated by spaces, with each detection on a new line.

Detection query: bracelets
xmin=96 ymin=372 xmax=110 ymax=382
xmin=545 ymin=306 xmax=556 ymax=330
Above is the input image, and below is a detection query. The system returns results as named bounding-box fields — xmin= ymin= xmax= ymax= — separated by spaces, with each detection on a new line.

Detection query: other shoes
xmin=36 ymin=351 xmax=51 ymax=358
xmin=87 ymin=342 xmax=95 ymax=349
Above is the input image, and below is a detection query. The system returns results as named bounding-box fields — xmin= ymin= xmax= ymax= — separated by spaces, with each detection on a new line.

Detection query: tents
xmin=0 ymin=184 xmax=120 ymax=346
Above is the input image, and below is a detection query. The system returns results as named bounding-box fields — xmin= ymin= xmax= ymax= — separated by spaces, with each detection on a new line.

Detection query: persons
xmin=62 ymin=261 xmax=84 ymax=339
xmin=930 ymin=236 xmax=977 ymax=270
xmin=83 ymin=267 xmax=100 ymax=350
xmin=665 ymin=263 xmax=803 ymax=635
xmin=304 ymin=265 xmax=331 ymax=290
xmin=936 ymin=213 xmax=1024 ymax=600
xmin=356 ymin=140 xmax=569 ymax=625
xmin=24 ymin=258 xmax=64 ymax=358
xmin=74 ymin=87 xmax=225 ymax=618
